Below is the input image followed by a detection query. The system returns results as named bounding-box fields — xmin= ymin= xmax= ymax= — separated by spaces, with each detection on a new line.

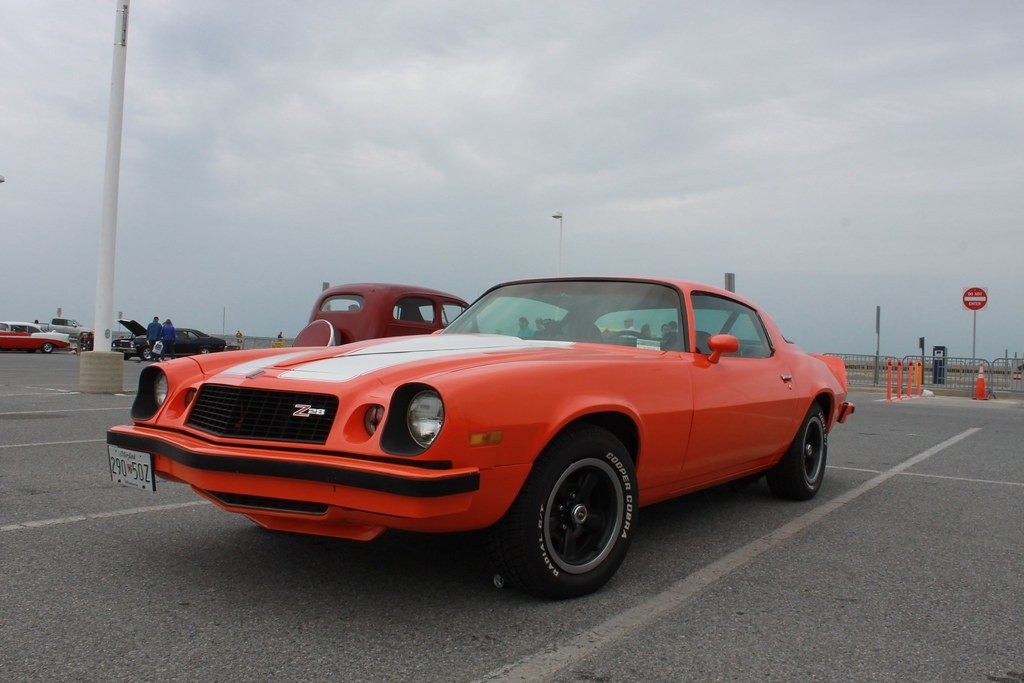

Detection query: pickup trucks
xmin=31 ymin=318 xmax=95 ymax=347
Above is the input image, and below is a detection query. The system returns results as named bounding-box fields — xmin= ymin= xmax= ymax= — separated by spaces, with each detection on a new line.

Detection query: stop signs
xmin=962 ymin=287 xmax=989 ymax=312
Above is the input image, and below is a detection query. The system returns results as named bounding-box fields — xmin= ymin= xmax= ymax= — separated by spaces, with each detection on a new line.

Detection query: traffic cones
xmin=973 ymin=362 xmax=990 ymax=401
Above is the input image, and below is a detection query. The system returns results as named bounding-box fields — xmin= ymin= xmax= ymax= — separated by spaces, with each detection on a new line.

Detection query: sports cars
xmin=107 ymin=275 xmax=855 ymax=604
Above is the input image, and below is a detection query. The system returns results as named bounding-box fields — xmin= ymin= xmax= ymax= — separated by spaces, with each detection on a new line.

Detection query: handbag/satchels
xmin=152 ymin=339 xmax=166 ymax=355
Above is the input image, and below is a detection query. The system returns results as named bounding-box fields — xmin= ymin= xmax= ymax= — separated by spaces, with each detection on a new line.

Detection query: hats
xmin=164 ymin=319 xmax=172 ymax=324
xmin=518 ymin=317 xmax=529 ymax=327
xmin=153 ymin=317 xmax=159 ymax=322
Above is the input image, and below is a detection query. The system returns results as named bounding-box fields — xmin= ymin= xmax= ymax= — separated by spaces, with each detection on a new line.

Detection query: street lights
xmin=551 ymin=214 xmax=565 ymax=273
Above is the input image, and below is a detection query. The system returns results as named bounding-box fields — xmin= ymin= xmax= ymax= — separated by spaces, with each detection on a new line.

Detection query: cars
xmin=110 ymin=319 xmax=227 ymax=362
xmin=288 ymin=282 xmax=484 ymax=346
xmin=0 ymin=321 xmax=71 ymax=353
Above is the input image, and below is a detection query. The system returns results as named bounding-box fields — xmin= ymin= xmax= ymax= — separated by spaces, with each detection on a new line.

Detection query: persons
xmin=517 ymin=317 xmax=545 ymax=338
xmin=77 ymin=331 xmax=93 ymax=351
xmin=146 ymin=317 xmax=177 ymax=362
xmin=622 ymin=318 xmax=636 ymax=337
xmin=236 ymin=331 xmax=242 ymax=349
xmin=278 ymin=332 xmax=284 ymax=342
xmin=661 ymin=320 xmax=677 ymax=345
